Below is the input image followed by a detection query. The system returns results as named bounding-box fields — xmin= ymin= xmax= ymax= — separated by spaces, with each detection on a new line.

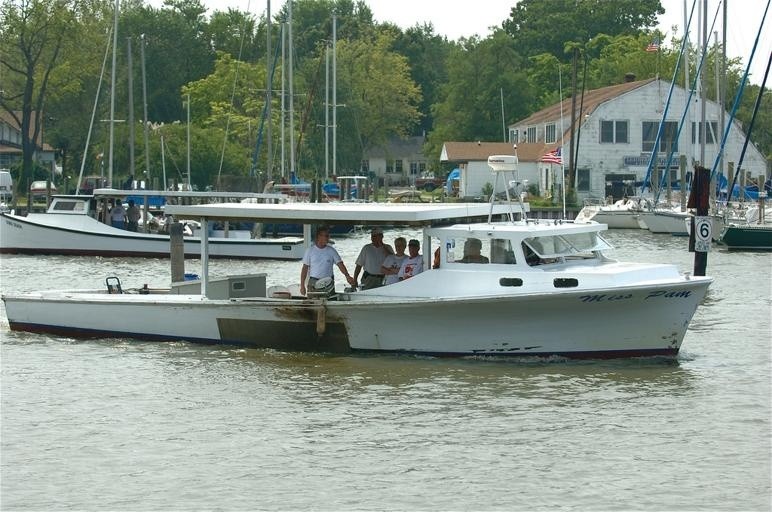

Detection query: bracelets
xmin=345 ymin=273 xmax=350 ymax=277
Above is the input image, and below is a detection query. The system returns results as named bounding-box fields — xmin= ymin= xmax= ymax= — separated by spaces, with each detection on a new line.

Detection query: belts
xmin=368 ymin=273 xmax=384 ymax=278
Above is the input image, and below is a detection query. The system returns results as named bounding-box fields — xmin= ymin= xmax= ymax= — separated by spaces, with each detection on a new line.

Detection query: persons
xmin=161 ymin=215 xmax=174 ymax=232
xmin=352 ymin=229 xmax=395 ymax=291
xmin=380 ymin=237 xmax=409 ymax=286
xmin=124 ymin=199 xmax=141 ymax=232
xmin=149 ymin=218 xmax=159 ymax=233
xmin=108 ymin=199 xmax=127 ymax=230
xmin=397 ymin=239 xmax=423 ymax=281
xmin=453 ymin=237 xmax=556 ymax=267
xmin=300 ymin=226 xmax=354 ymax=302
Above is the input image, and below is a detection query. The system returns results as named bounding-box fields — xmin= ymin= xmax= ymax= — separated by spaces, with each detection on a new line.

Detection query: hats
xmin=371 ymin=227 xmax=385 ymax=235
xmin=408 ymin=239 xmax=421 ymax=247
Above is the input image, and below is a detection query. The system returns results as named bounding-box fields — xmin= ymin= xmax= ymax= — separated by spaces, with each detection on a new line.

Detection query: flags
xmin=538 ymin=147 xmax=563 ymax=166
xmin=646 ymin=44 xmax=659 ymax=52
